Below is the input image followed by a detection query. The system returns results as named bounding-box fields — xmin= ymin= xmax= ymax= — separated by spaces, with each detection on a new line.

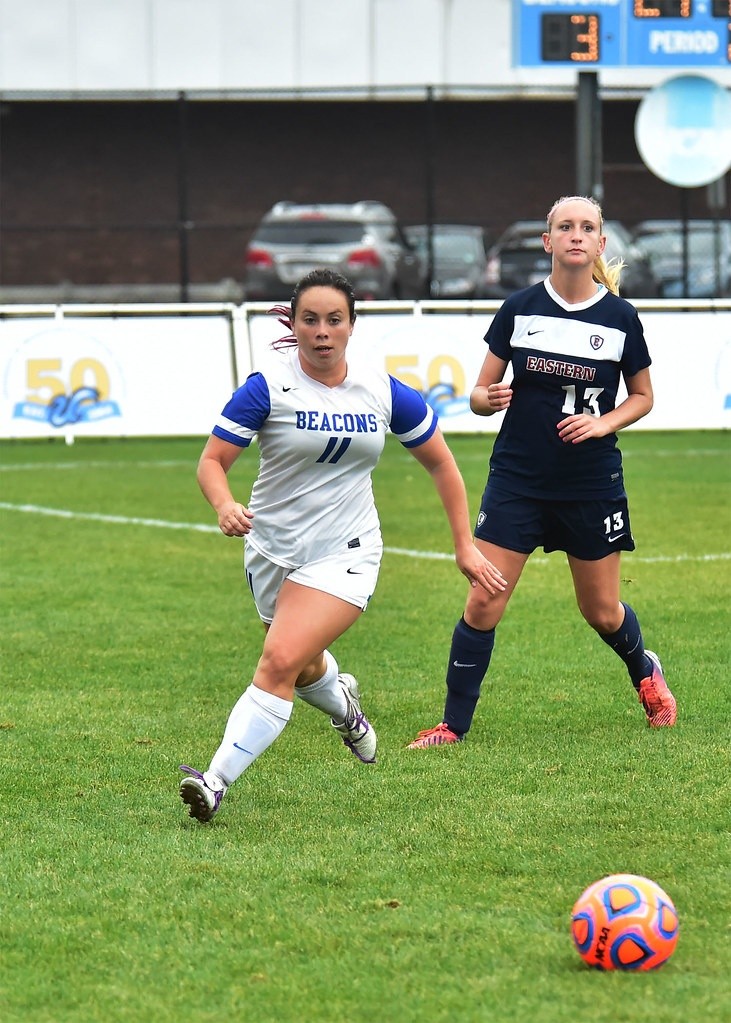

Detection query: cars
xmin=398 ymin=218 xmax=496 ymax=298
xmin=485 ymin=219 xmax=643 ymax=297
xmin=622 ymin=219 xmax=731 ymax=300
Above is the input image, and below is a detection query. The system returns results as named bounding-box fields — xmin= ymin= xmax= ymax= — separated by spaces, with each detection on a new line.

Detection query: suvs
xmin=245 ymin=196 xmax=421 ymax=301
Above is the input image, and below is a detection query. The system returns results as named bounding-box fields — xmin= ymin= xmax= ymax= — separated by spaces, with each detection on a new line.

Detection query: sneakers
xmin=331 ymin=672 xmax=376 ymax=763
xmin=634 ymin=650 xmax=677 ymax=728
xmin=406 ymin=726 xmax=459 ymax=747
xmin=180 ymin=772 xmax=224 ymax=826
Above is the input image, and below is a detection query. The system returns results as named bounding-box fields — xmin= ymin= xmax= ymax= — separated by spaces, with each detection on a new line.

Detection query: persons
xmin=181 ymin=270 xmax=510 ymax=821
xmin=407 ymin=193 xmax=676 ymax=749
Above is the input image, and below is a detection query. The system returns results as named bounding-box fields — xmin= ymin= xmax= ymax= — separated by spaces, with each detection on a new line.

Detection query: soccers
xmin=570 ymin=873 xmax=682 ymax=971
